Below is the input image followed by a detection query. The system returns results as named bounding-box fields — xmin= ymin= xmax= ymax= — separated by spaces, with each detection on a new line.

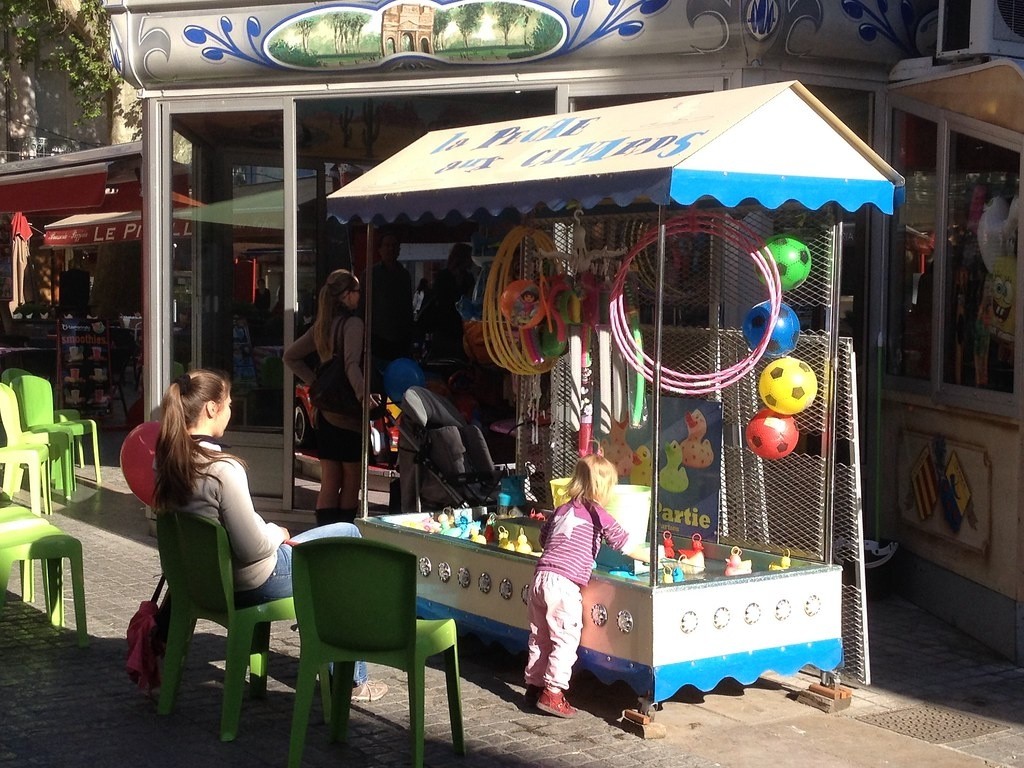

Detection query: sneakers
xmin=351 ymin=680 xmax=388 ymax=702
xmin=525 ymin=683 xmax=543 ymax=704
xmin=536 ymin=686 xmax=578 ymax=719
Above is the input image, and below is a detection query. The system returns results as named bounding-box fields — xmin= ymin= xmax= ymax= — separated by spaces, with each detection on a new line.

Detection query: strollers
xmin=369 ymin=385 xmax=506 ymax=515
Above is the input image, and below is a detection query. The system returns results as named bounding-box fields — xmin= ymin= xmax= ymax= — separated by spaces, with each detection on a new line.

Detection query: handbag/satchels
xmin=307 ymin=313 xmax=388 ymax=421
xmin=128 ymin=603 xmax=165 ymax=684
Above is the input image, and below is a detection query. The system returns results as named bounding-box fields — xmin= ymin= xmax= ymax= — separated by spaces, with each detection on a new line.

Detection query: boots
xmin=315 ymin=506 xmax=359 ymax=527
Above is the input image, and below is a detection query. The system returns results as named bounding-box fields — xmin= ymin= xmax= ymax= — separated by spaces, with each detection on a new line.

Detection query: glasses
xmin=344 ymin=287 xmax=364 ymax=296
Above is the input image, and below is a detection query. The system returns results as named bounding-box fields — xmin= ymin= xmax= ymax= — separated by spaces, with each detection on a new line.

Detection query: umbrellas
xmin=10 ymin=212 xmax=33 ymax=318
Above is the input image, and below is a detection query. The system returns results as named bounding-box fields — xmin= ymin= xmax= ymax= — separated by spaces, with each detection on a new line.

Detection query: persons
xmin=361 ymin=234 xmax=414 ymax=361
xmin=441 ymin=241 xmax=477 ymax=299
xmin=248 ymin=280 xmax=271 ymax=310
xmin=282 ymin=267 xmax=379 ymax=529
xmin=148 ymin=370 xmax=388 ymax=702
xmin=523 ymin=454 xmax=651 ymax=719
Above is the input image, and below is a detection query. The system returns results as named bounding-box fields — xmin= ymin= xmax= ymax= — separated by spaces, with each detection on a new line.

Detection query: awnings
xmin=0 ymin=162 xmax=112 ymax=213
xmin=41 ymin=205 xmax=193 ymax=247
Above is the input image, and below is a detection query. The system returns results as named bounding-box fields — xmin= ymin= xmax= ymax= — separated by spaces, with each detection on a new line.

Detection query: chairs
xmin=0 ymin=367 xmax=101 ymax=517
xmin=152 ymin=510 xmax=331 ymax=742
xmin=288 ymin=537 xmax=467 ymax=768
xmin=0 ymin=499 xmax=90 ymax=647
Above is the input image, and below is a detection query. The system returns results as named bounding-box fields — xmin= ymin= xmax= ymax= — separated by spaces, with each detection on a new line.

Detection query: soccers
xmin=755 ymin=232 xmax=813 ymax=291
xmin=756 ymin=355 xmax=819 ymax=415
xmin=744 ymin=299 xmax=800 ymax=358
xmin=746 ymin=408 xmax=799 ymax=459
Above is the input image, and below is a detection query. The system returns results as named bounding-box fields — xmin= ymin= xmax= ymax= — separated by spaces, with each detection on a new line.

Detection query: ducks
xmin=421 ymin=506 xmax=791 ymax=584
xmin=600 ymin=408 xmax=714 ymax=493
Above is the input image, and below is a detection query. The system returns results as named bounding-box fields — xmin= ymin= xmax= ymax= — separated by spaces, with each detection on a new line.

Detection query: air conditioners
xmin=936 ymin=0 xmax=1024 ymax=59
